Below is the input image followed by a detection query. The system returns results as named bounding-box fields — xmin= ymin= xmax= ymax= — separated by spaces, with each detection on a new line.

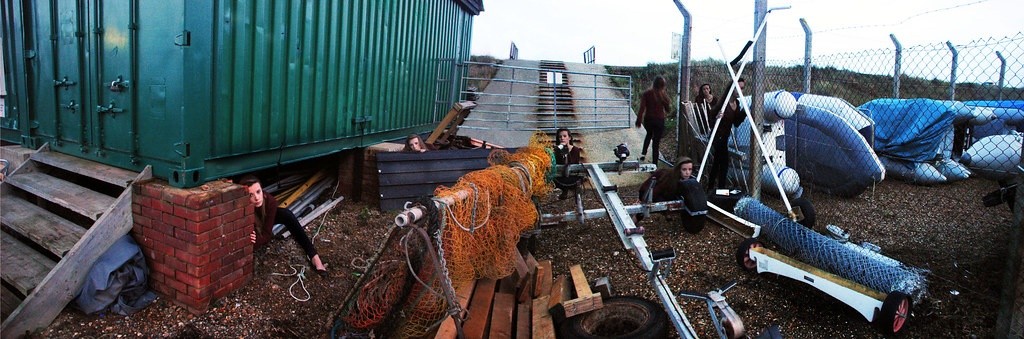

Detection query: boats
xmin=786 ymin=92 xmax=885 ymax=198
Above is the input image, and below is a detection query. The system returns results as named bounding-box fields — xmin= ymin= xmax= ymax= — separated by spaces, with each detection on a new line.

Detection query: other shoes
xmin=309 ymin=261 xmax=328 ymax=279
xmin=637 ymin=157 xmax=645 ymax=161
xmin=665 ymin=216 xmax=673 ymax=222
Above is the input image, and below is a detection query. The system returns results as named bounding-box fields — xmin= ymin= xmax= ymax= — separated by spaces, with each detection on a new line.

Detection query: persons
xmin=402 ymin=133 xmax=430 ymax=153
xmin=694 ymin=84 xmax=718 ymax=135
xmin=632 ymin=156 xmax=694 ymax=225
xmin=635 ymin=75 xmax=672 ymax=164
xmin=551 ymin=128 xmax=582 ymax=200
xmin=241 ymin=179 xmax=327 ymax=276
xmin=707 ymin=78 xmax=750 ymax=191
